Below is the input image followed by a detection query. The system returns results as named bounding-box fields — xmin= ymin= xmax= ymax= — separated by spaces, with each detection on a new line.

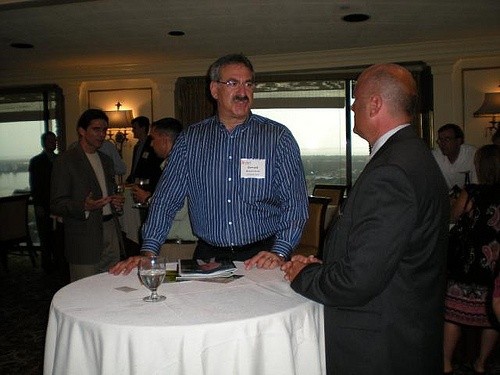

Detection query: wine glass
xmin=139 ymin=255 xmax=167 ymax=302
xmin=113 ymin=182 xmax=125 ymax=207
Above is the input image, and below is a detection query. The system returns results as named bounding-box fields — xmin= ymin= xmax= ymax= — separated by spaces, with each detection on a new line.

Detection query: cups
xmin=134 ymin=178 xmax=150 ymax=208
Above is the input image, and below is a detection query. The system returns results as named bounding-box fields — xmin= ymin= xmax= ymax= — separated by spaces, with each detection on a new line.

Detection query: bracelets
xmin=276 ymin=253 xmax=284 ymax=257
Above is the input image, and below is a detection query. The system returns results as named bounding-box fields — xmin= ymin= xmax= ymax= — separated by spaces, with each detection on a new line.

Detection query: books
xmin=178 ymin=259 xmax=238 ymax=278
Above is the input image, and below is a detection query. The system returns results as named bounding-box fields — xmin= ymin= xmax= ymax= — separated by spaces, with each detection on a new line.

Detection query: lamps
xmin=474 ymin=92 xmax=500 ymax=137
xmin=104 ymin=102 xmax=133 ymax=143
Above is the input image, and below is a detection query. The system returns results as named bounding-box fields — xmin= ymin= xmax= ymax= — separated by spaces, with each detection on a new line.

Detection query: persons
xmin=281 ymin=63 xmax=452 ymax=374
xmin=132 ymin=117 xmax=197 ymax=259
xmin=441 ymin=145 xmax=499 ymax=373
xmin=430 ymin=124 xmax=480 ymax=188
xmin=28 ymin=132 xmax=60 ymax=263
xmin=181 ymin=259 xmax=224 ymax=274
xmin=49 ymin=108 xmax=125 ymax=281
xmin=110 ymin=53 xmax=309 ymax=277
xmin=67 ymin=139 xmax=126 ymax=176
xmin=123 ymin=116 xmax=157 ymax=195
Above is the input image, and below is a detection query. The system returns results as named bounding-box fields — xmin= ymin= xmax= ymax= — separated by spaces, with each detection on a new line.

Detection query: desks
xmin=43 ymin=261 xmax=325 ymax=375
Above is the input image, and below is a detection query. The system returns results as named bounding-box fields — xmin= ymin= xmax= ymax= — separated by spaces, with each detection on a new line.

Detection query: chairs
xmin=313 ymin=184 xmax=348 ymax=205
xmin=290 ymin=195 xmax=332 ymax=259
xmin=0 ymin=194 xmax=32 ymax=270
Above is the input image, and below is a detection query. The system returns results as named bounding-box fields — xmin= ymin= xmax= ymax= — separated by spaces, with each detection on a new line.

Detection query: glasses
xmin=436 ymin=136 xmax=458 ymax=144
xmin=216 ymin=79 xmax=253 ymax=88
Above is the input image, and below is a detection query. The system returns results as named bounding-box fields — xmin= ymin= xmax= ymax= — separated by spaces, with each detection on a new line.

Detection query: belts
xmin=199 ymin=234 xmax=276 ymax=253
xmin=164 ymin=238 xmax=198 ymax=245
xmin=103 ymin=213 xmax=114 ymax=223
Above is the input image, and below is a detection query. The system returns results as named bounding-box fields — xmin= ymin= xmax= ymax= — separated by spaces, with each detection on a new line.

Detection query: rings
xmin=269 ymin=257 xmax=273 ymax=261
